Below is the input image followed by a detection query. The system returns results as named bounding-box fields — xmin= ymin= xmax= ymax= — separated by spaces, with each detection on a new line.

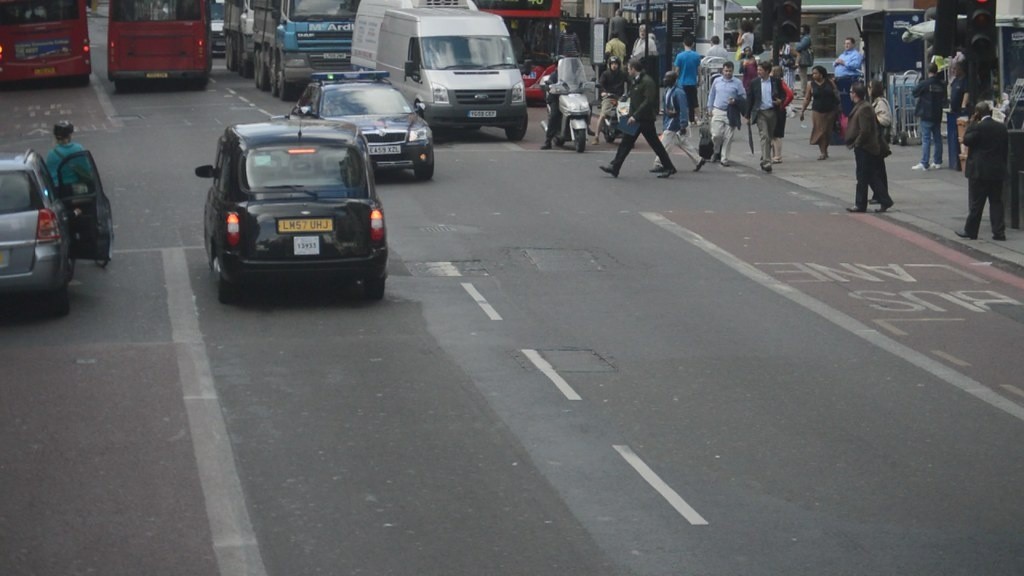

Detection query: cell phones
xmin=974 ymin=111 xmax=980 ymax=121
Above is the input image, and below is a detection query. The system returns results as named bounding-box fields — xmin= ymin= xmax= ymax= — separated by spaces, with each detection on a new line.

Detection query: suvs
xmin=0 ymin=146 xmax=116 ymax=320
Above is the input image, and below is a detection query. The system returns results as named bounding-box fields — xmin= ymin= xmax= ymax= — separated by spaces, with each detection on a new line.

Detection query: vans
xmin=372 ymin=7 xmax=530 ymax=145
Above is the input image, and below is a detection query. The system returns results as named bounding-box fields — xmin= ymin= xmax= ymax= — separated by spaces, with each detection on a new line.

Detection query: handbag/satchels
xmin=880 ymin=126 xmax=892 ymax=143
xmin=799 ymin=48 xmax=814 ymax=67
xmin=837 ymin=111 xmax=848 ymax=139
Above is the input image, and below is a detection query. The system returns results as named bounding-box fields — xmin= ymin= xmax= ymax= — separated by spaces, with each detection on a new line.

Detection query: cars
xmin=288 ymin=69 xmax=435 ymax=184
xmin=193 ymin=115 xmax=390 ymax=306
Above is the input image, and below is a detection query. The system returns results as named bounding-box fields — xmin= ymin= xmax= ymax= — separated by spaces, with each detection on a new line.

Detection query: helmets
xmin=553 ymin=55 xmax=564 ymax=63
xmin=608 ymin=56 xmax=621 ymax=68
xmin=54 ymin=121 xmax=73 ymax=135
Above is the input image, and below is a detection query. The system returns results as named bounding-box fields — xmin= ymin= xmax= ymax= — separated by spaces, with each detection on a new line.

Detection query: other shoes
xmin=599 ymin=165 xmax=618 ymax=177
xmin=694 ymin=158 xmax=705 ymax=171
xmin=818 ymin=154 xmax=825 ymax=160
xmin=869 ymin=199 xmax=878 ymax=204
xmin=760 ymin=163 xmax=772 ymax=171
xmin=710 ymin=153 xmax=718 ymax=162
xmin=847 ymin=206 xmax=859 ymax=211
xmin=657 ymin=168 xmax=677 ymax=178
xmin=954 ymin=230 xmax=977 ymax=239
xmin=875 ymin=203 xmax=893 ymax=212
xmin=773 ymin=159 xmax=782 ymax=163
xmin=588 ymin=129 xmax=595 ymax=136
xmin=912 ymin=163 xmax=925 ymax=170
xmin=928 ymin=163 xmax=941 ymax=169
xmin=721 ymin=161 xmax=730 ymax=166
xmin=992 ymin=235 xmax=1005 ymax=240
xmin=650 ymin=164 xmax=662 ymax=171
xmin=541 ymin=144 xmax=552 ymax=149
xmin=592 ymin=136 xmax=599 ymax=144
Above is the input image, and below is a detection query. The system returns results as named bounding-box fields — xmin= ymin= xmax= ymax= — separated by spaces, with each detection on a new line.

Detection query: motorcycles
xmin=539 ymin=57 xmax=594 ymax=154
xmin=593 ymin=80 xmax=633 ymax=144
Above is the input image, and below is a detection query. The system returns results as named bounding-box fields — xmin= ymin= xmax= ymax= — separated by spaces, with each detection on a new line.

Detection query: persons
xmin=832 ymin=38 xmax=863 ymax=117
xmin=845 ymin=79 xmax=894 ymax=213
xmin=707 ymin=21 xmax=792 ymax=172
xmin=910 ymin=63 xmax=946 ymax=171
xmin=946 ymin=61 xmax=972 ymax=111
xmin=559 ymin=25 xmax=583 ymax=57
xmin=592 ymin=9 xmax=706 ymax=179
xmin=795 ymin=24 xmax=814 ymax=98
xmin=541 ymin=58 xmax=596 ymax=150
xmin=954 ymin=103 xmax=1008 ymax=241
xmin=799 ymin=66 xmax=843 ymax=161
xmin=44 ymin=122 xmax=94 ymax=188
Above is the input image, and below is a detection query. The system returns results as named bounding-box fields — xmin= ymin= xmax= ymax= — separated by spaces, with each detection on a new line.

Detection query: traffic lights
xmin=965 ymin=0 xmax=997 ymax=67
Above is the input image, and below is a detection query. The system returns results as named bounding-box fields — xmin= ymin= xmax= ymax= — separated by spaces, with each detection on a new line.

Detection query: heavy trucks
xmin=212 ymin=0 xmax=364 ymax=102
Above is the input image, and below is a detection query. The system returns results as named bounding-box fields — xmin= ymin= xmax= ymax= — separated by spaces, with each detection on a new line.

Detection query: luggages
xmin=698 ymin=112 xmax=721 ymax=160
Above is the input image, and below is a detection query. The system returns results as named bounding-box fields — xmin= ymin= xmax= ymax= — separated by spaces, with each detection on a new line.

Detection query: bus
xmin=471 ymin=0 xmax=571 ymax=106
xmin=0 ymin=0 xmax=93 ymax=90
xmin=105 ymin=0 xmax=215 ymax=91
